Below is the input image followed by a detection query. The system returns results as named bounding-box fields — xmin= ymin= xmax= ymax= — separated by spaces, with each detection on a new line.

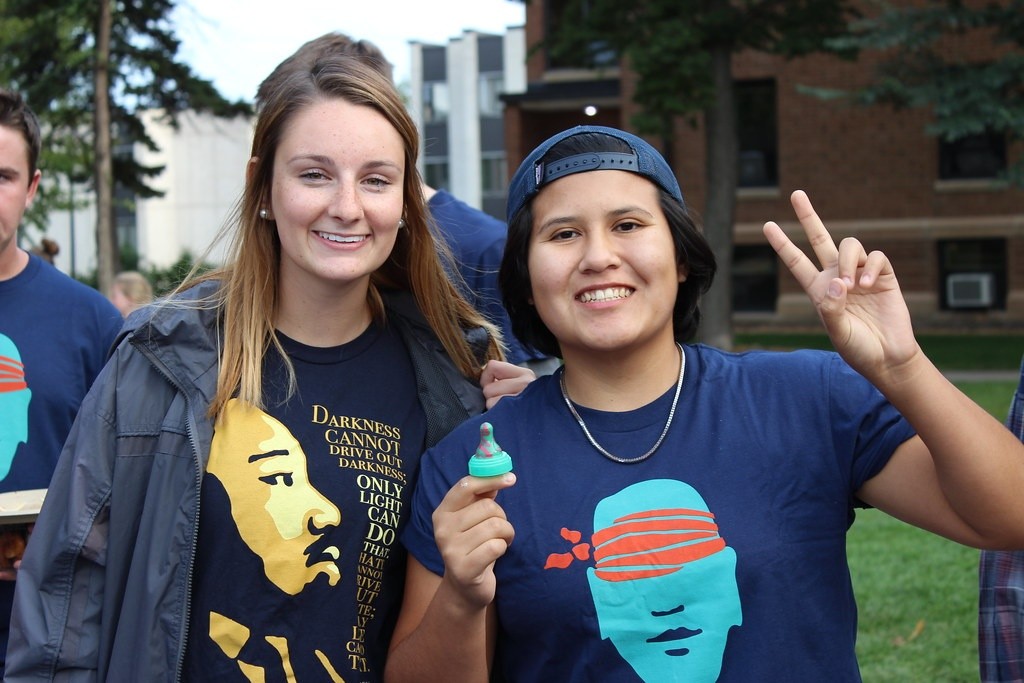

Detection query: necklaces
xmin=560 ymin=341 xmax=686 ymax=463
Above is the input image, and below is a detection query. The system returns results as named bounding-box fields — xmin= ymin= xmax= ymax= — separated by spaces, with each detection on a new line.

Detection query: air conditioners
xmin=948 ymin=272 xmax=996 ymax=307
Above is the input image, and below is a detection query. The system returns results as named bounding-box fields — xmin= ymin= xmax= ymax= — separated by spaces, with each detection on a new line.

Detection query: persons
xmin=0 ymin=56 xmax=536 ymax=683
xmin=254 ymin=30 xmax=562 ymax=380
xmin=107 ymin=270 xmax=152 ymax=318
xmin=383 ymin=124 xmax=1024 ymax=683
xmin=0 ymin=89 xmax=124 ymax=683
xmin=979 ymin=362 xmax=1024 ymax=683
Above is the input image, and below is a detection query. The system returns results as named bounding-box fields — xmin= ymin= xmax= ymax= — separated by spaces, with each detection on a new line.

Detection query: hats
xmin=506 ymin=125 xmax=688 ymax=227
